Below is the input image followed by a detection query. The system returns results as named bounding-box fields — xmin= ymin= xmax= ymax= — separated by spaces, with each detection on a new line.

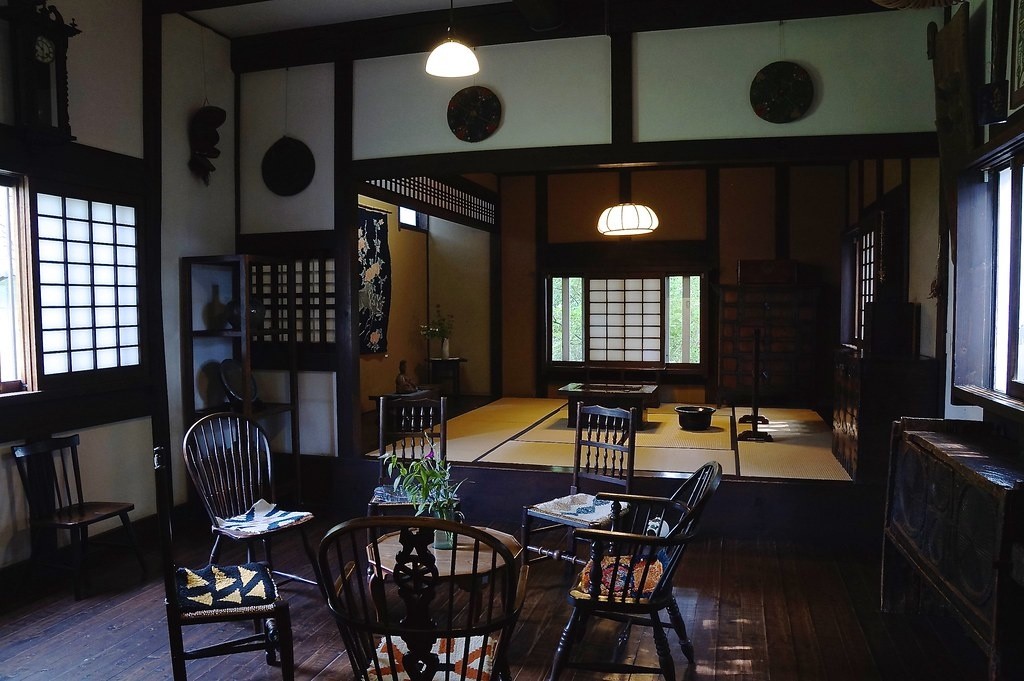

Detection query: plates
xmin=219 ymin=358 xmax=258 ymax=406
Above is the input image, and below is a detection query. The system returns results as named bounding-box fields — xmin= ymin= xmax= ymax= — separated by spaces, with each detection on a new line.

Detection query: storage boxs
xmin=864 ymin=301 xmax=915 ymax=352
xmin=736 ymin=258 xmax=800 ymax=286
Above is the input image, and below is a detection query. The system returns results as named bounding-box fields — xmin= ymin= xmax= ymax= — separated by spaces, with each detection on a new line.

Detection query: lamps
xmin=596 ymin=199 xmax=662 ymax=240
xmin=425 ymin=0 xmax=480 ymax=78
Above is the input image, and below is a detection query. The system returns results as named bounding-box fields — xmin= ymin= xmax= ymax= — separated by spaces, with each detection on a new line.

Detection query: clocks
xmin=0 ymin=0 xmax=82 ymax=146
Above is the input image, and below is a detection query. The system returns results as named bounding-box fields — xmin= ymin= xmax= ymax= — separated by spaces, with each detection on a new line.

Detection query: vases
xmin=432 ymin=503 xmax=467 ymax=552
xmin=439 ymin=337 xmax=452 ymax=360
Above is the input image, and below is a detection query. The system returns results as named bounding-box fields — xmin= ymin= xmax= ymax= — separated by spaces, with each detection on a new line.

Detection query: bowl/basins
xmin=674 ymin=406 xmax=717 ymax=430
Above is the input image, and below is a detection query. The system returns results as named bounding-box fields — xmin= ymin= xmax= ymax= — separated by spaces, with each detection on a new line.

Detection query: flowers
xmin=416 ymin=303 xmax=458 ymax=339
xmin=377 ymin=440 xmax=478 ymax=540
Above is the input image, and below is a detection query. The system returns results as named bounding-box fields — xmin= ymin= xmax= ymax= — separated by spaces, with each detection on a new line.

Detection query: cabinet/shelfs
xmin=179 ymin=254 xmax=303 ymax=508
xmin=711 ymin=283 xmax=823 ymax=408
xmin=831 ymin=350 xmax=939 ymax=484
xmin=878 ymin=414 xmax=1024 ymax=681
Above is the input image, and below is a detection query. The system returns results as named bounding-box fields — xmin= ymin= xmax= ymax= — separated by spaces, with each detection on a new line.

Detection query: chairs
xmin=316 ymin=514 xmax=531 ymax=681
xmin=153 ymin=446 xmax=295 ymax=681
xmin=11 ymin=434 xmax=151 ymax=602
xmin=521 ymin=400 xmax=638 ymax=581
xmin=546 ymin=461 xmax=724 ymax=681
xmin=366 ymin=391 xmax=450 ymax=544
xmin=181 ymin=410 xmax=328 ymax=634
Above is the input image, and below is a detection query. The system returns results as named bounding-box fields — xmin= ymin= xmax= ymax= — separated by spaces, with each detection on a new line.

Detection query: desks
xmin=557 ymin=382 xmax=660 ymax=430
xmin=424 ymin=357 xmax=467 ymax=396
xmin=369 ymin=384 xmax=443 ymax=424
xmin=364 ymin=525 xmax=526 ymax=625
